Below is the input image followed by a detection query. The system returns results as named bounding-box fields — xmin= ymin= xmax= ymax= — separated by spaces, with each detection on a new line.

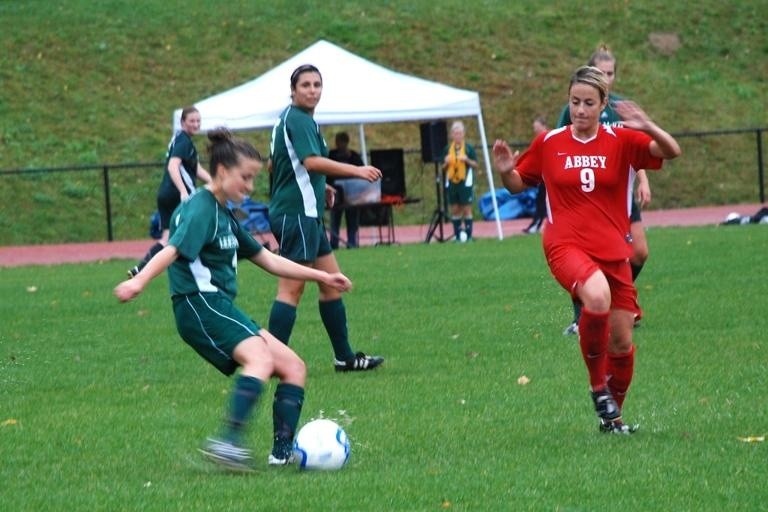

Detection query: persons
xmin=555 ymin=41 xmax=652 ymax=338
xmin=519 ymin=116 xmax=549 ymax=235
xmin=266 ymin=64 xmax=385 ymax=372
xmin=111 ymin=124 xmax=352 ymax=468
xmin=324 ymin=129 xmax=364 ymax=248
xmin=442 ymin=120 xmax=479 ymax=244
xmin=490 ymin=64 xmax=682 ymax=434
xmin=125 ymin=105 xmax=211 ymax=278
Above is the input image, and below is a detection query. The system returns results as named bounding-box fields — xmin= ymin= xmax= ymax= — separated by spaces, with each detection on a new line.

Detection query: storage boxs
xmin=332 ymin=177 xmax=383 ymax=205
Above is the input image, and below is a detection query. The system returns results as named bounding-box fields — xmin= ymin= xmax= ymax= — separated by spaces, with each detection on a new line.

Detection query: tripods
xmin=424 ymin=164 xmax=456 ymax=244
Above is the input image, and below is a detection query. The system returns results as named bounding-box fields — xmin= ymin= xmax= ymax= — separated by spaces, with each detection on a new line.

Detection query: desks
xmin=246 ymin=195 xmax=421 ymax=246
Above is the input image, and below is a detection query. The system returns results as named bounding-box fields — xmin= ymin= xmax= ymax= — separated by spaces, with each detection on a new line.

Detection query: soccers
xmin=295 ymin=417 xmax=349 ymax=472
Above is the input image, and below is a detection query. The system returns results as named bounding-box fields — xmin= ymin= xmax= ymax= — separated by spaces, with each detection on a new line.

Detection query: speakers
xmin=370 ymin=148 xmax=405 ymax=196
xmin=420 ymin=122 xmax=448 ymax=163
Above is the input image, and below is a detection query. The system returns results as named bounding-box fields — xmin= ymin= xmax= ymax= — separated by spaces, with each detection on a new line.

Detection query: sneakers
xmin=588 ymin=384 xmax=640 ymax=434
xmin=334 ymin=352 xmax=384 ymax=372
xmin=268 ymin=453 xmax=296 ymax=466
